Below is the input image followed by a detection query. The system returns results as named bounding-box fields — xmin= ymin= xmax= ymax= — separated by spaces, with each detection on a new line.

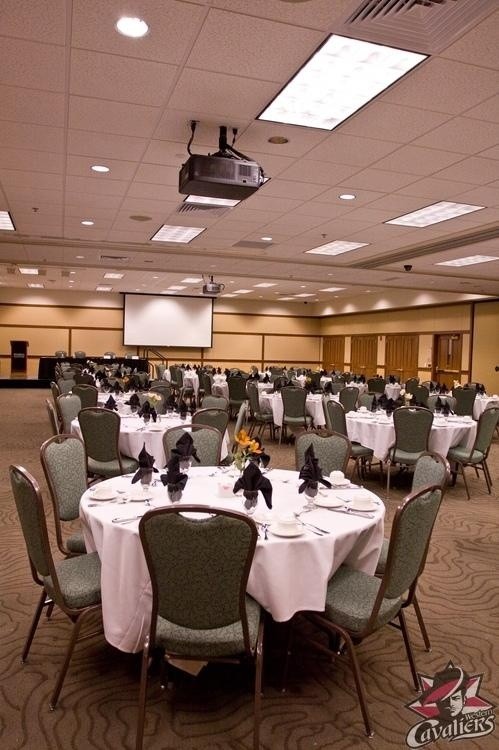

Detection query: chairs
xmin=9 ymin=365 xmax=499 ymax=750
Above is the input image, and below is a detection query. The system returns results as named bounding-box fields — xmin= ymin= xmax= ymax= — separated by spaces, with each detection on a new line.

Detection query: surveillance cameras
xmin=404 ymin=265 xmax=413 ymax=271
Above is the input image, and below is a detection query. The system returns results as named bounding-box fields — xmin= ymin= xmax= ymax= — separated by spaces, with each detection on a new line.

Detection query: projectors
xmin=203 ymin=283 xmax=221 ymax=294
xmin=178 ymin=153 xmax=263 ymax=201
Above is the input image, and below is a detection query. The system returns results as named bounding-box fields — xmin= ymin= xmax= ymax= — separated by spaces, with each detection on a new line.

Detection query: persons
xmin=246 ymin=365 xmax=260 ymax=381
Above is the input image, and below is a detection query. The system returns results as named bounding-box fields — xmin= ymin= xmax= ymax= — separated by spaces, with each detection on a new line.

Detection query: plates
xmin=344 ymin=405 xmax=474 ymax=427
xmin=88 ymin=456 xmax=381 ymax=539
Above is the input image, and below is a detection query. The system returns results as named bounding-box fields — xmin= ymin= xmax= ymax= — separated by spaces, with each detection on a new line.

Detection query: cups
xmin=261 ymin=388 xmax=339 ymax=401
xmin=97 ymin=390 xmax=194 ymax=433
xmin=428 ymin=385 xmax=498 ymax=400
